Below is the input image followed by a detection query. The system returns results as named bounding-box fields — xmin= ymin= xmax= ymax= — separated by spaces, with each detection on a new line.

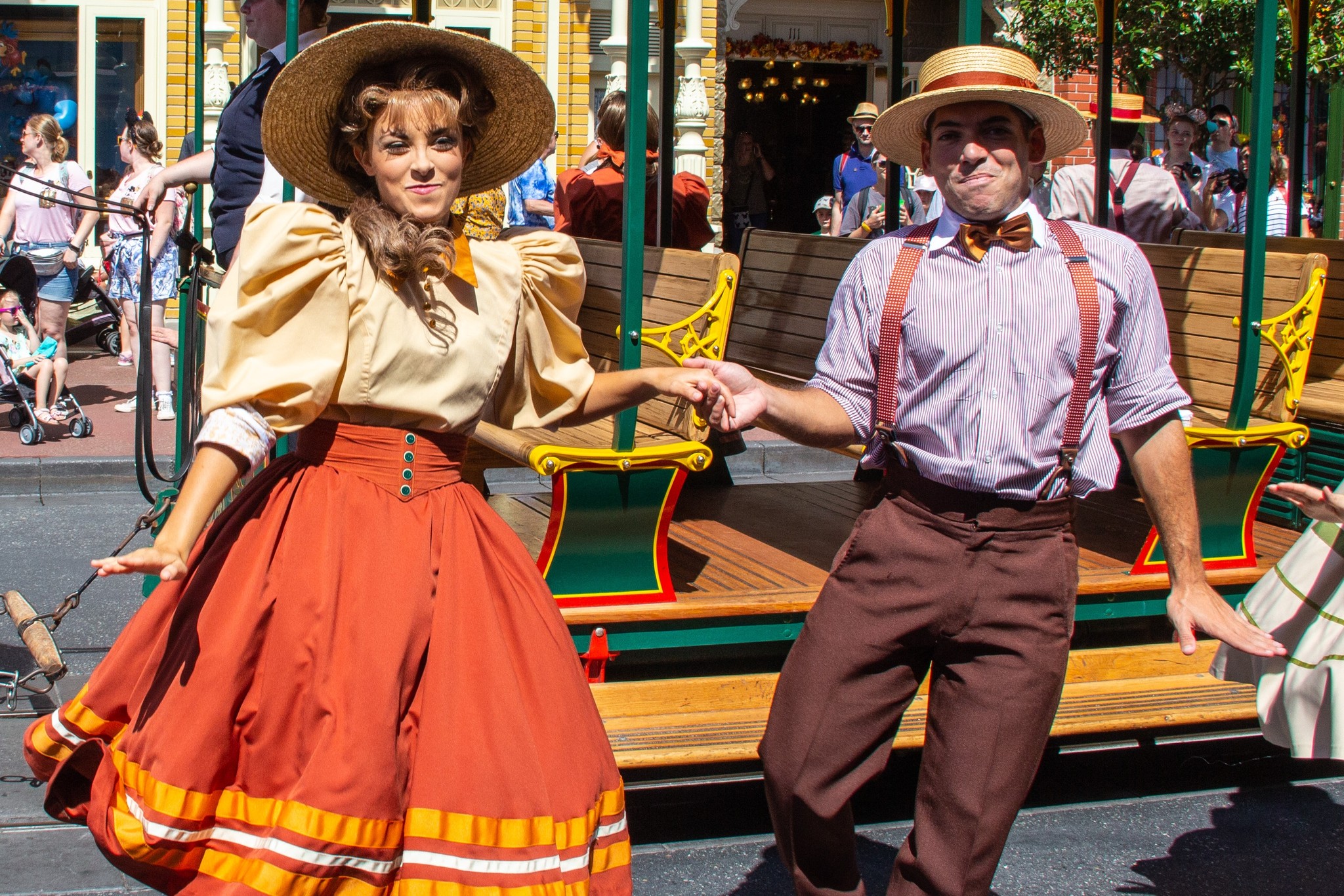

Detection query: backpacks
xmin=20 ymin=160 xmax=86 ymax=258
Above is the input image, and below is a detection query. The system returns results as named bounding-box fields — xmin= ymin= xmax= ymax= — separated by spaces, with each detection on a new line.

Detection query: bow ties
xmin=957 ymin=212 xmax=1034 ymax=263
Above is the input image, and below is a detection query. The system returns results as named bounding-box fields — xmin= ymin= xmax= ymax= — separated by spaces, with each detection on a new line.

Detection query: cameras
xmin=1223 ymin=167 xmax=1248 ymax=193
xmin=1176 ymin=160 xmax=1203 ymax=182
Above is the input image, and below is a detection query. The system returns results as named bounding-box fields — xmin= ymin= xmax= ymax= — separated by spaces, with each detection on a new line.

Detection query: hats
xmin=871 ymin=44 xmax=1088 ymax=167
xmin=259 ymin=19 xmax=557 ymax=209
xmin=847 ymin=102 xmax=878 ymax=125
xmin=1077 ymin=93 xmax=1161 ymax=123
xmin=812 ymin=196 xmax=834 ymax=213
xmin=1207 ymin=105 xmax=1239 ymax=131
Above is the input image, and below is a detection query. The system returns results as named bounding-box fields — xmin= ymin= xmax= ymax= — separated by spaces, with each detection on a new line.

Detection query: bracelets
xmin=0 ymin=235 xmax=5 ymax=240
xmin=860 ymin=220 xmax=873 ymax=232
xmin=150 ymin=257 xmax=157 ymax=268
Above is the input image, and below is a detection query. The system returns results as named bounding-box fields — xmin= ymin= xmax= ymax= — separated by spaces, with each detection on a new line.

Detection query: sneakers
xmin=155 ymin=396 xmax=176 ymax=420
xmin=114 ymin=394 xmax=156 ymax=413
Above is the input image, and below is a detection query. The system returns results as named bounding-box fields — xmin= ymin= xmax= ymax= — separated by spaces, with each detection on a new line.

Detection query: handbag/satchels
xmin=732 ymin=205 xmax=751 ymax=228
xmin=16 ymin=247 xmax=66 ymax=274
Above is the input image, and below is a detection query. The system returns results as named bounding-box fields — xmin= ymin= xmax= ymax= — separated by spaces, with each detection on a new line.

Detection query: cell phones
xmin=752 ymin=147 xmax=756 ymax=155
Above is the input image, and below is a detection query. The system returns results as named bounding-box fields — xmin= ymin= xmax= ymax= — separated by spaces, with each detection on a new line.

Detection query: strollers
xmin=2 ymin=253 xmax=94 ymax=445
xmin=66 ymin=261 xmax=122 ymax=357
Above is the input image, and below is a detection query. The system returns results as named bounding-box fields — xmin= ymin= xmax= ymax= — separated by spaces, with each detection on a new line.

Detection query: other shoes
xmin=117 ymin=352 xmax=133 ymax=366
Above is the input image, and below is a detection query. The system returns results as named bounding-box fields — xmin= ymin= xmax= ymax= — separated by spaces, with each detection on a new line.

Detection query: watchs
xmin=68 ymin=243 xmax=83 ymax=253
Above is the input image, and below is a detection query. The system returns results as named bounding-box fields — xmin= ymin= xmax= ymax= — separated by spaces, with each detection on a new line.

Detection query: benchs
xmin=467 ymin=225 xmax=1344 ymax=612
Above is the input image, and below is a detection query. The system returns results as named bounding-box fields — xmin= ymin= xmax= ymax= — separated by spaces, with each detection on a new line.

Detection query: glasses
xmin=853 ymin=124 xmax=872 ymax=133
xmin=0 ymin=306 xmax=24 ymax=315
xmin=22 ymin=129 xmax=47 ymax=142
xmin=117 ymin=136 xmax=136 ymax=149
xmin=1210 ymin=119 xmax=1233 ymax=129
xmin=553 ymin=131 xmax=559 ymax=140
xmin=872 ymin=160 xmax=886 ymax=168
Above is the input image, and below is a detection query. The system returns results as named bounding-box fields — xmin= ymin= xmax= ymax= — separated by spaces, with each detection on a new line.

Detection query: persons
xmin=131 ymin=1 xmax=328 ymax=270
xmin=1027 ymin=90 xmax=1329 ymax=246
xmin=90 ymin=270 xmax=109 ymax=288
xmin=1210 ymin=482 xmax=1344 ymax=760
xmin=118 ymin=313 xmax=136 ymax=366
xmin=0 ymin=285 xmax=69 ymax=425
xmin=682 ymin=43 xmax=1287 ymax=896
xmin=723 ymin=132 xmax=778 ymax=253
xmin=177 ymin=81 xmax=237 ymax=219
xmin=150 ymin=326 xmax=181 ymax=349
xmin=0 ymin=114 xmax=101 ymax=362
xmin=101 ymin=107 xmax=180 ymax=421
xmin=811 ymin=101 xmax=943 ymax=239
xmin=553 ymin=90 xmax=716 ymax=250
xmin=506 ymin=128 xmax=559 ymax=231
xmin=27 ymin=20 xmax=736 ymax=896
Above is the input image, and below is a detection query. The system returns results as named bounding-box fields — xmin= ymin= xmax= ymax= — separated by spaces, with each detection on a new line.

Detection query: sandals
xmin=33 ymin=407 xmax=59 ymax=425
xmin=48 ymin=405 xmax=65 ymax=420
